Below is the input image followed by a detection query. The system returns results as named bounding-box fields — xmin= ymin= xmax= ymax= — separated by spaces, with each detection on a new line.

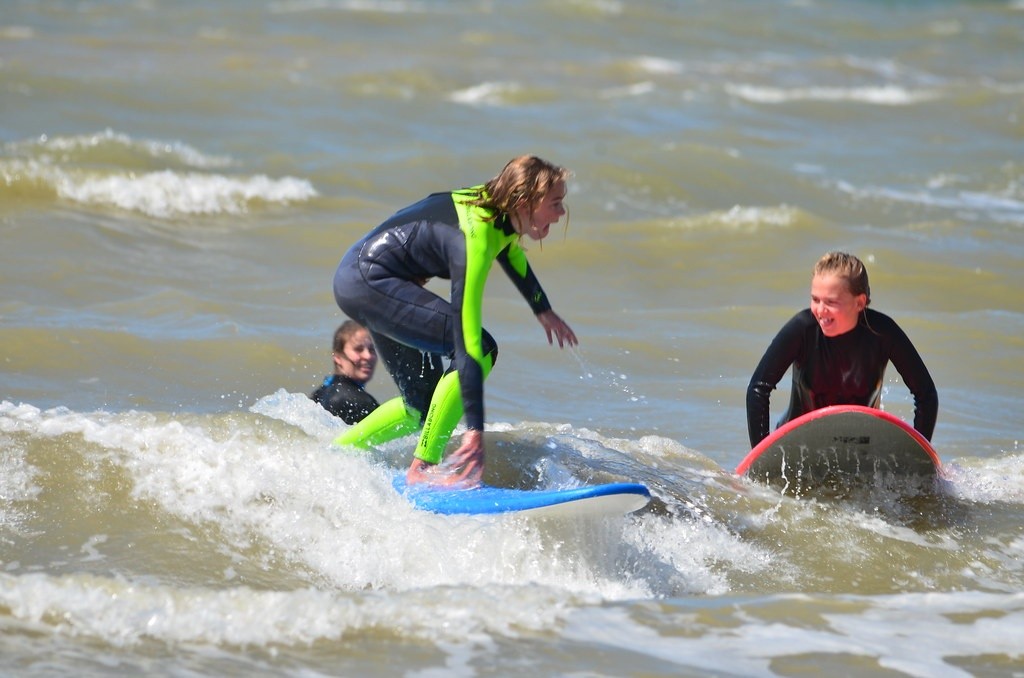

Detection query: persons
xmin=309 ymin=320 xmax=380 ymax=428
xmin=332 ymin=154 xmax=580 ymax=493
xmin=745 ymin=251 xmax=939 ymax=450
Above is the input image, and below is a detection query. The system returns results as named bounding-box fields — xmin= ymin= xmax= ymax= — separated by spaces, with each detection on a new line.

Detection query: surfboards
xmin=735 ymin=404 xmax=942 ymax=476
xmin=385 ymin=466 xmax=655 ymax=525
